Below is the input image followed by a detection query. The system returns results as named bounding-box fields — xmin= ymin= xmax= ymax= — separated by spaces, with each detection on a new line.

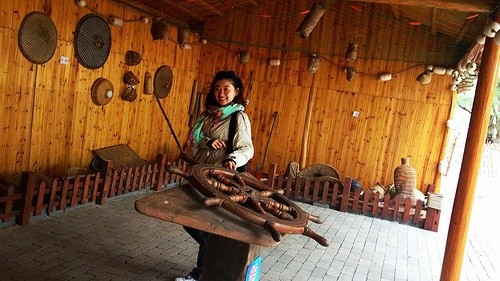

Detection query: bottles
xmin=362 ymin=182 xmax=387 ymax=203
xmin=394 ymin=157 xmax=417 ymax=199
xmin=146 ymin=72 xmax=152 ymax=94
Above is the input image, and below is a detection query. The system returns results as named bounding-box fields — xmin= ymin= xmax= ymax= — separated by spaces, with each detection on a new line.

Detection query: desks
xmin=135 ymin=184 xmax=293 ymax=281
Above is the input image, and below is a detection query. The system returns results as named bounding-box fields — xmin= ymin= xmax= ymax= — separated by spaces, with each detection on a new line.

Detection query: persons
xmin=173 ymin=70 xmax=254 ymax=281
